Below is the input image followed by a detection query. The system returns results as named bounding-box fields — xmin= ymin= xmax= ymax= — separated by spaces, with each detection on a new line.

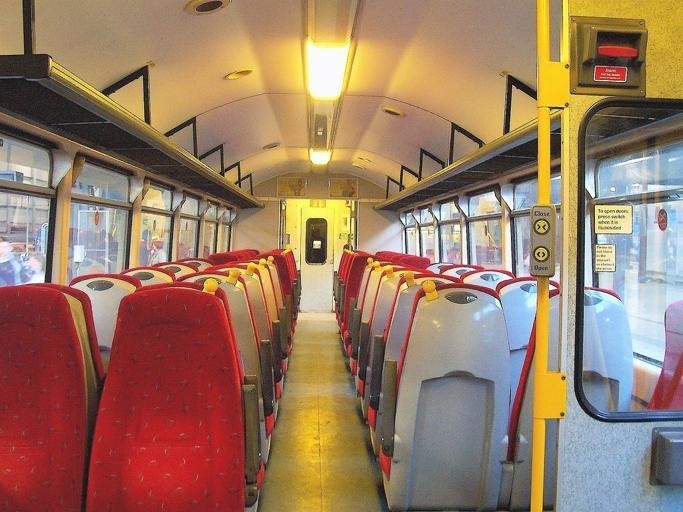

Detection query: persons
xmin=147 ymin=241 xmax=169 ymax=266
xmin=0 ymin=241 xmax=21 ymax=285
xmin=25 ymin=254 xmax=45 ymax=284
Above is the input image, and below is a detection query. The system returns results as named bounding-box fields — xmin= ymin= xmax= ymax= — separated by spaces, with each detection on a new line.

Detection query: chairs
xmin=331 ymin=250 xmax=682 ymax=511
xmin=0 ymin=249 xmax=302 ymax=511
